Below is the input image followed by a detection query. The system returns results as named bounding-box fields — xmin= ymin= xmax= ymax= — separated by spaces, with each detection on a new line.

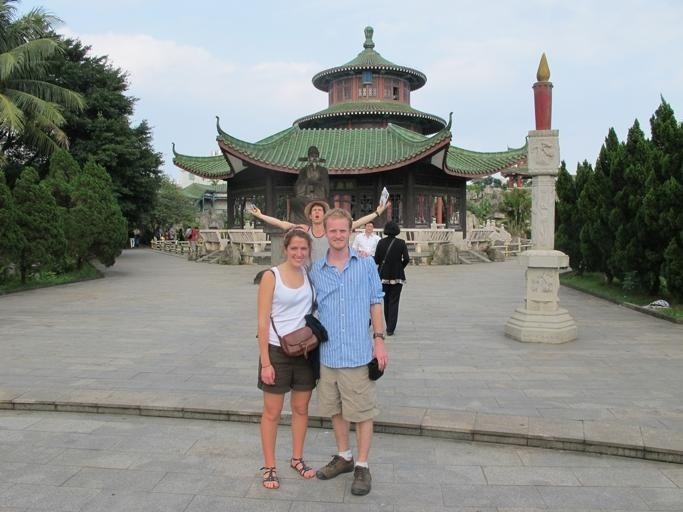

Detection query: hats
xmin=387 ymin=330 xmax=393 ymax=335
xmin=384 ymin=222 xmax=400 ymax=235
xmin=303 ymin=200 xmax=330 ymax=224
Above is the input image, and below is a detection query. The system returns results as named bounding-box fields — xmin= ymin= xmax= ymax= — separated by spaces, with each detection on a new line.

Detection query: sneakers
xmin=315 ymin=455 xmax=354 ymax=479
xmin=351 ymin=465 xmax=372 ymax=495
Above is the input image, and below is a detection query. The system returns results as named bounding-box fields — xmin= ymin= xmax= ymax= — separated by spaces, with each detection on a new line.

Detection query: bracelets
xmin=371 ymin=332 xmax=385 ymax=340
xmin=260 ymin=364 xmax=271 ymax=369
xmin=374 ymin=210 xmax=380 ymax=218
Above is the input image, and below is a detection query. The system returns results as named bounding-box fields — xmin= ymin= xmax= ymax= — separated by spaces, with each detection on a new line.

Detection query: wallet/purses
xmin=368 ymin=358 xmax=383 ymax=380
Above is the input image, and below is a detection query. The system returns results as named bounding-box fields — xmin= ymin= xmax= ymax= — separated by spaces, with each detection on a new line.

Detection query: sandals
xmin=290 ymin=457 xmax=315 ymax=479
xmin=260 ymin=466 xmax=280 ymax=489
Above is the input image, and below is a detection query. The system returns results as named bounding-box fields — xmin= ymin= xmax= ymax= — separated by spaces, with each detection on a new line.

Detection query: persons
xmin=252 ymin=225 xmax=323 ymax=490
xmin=126 ymin=220 xmax=200 ymax=252
xmin=372 ymin=219 xmax=410 ymax=336
xmin=288 ymin=145 xmax=332 ymax=226
xmin=306 ymin=208 xmax=388 ymax=497
xmin=245 ymin=197 xmax=393 ymax=273
xmin=351 ymin=220 xmax=383 ymax=261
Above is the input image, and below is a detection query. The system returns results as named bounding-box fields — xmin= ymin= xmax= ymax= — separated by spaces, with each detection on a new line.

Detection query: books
xmin=378 ymin=185 xmax=390 ymax=206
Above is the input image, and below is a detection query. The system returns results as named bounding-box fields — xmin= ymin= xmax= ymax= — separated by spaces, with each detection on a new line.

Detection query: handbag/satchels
xmin=280 ymin=326 xmax=321 ymax=357
